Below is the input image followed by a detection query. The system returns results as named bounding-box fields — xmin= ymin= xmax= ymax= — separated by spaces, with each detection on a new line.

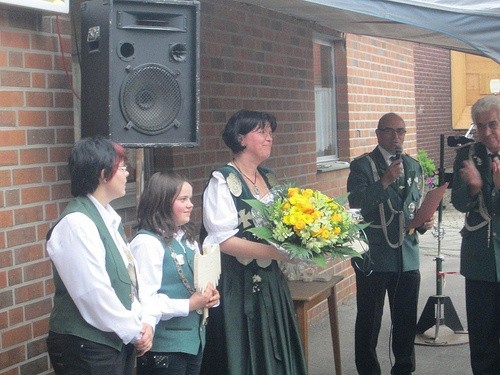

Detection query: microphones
xmin=395 ymin=147 xmax=403 ymax=186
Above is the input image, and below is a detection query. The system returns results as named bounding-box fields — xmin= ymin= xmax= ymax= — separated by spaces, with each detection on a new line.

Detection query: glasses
xmin=253 ymin=129 xmax=274 ymax=137
xmin=378 ymin=128 xmax=406 ymax=134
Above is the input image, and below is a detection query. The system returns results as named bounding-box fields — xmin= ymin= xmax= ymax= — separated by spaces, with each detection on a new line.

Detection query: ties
xmin=389 ymin=156 xmax=405 ymax=192
xmin=489 ymin=152 xmax=500 ymax=192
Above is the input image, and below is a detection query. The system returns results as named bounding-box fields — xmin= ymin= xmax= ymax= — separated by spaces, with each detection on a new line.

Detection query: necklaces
xmin=232 ymin=158 xmax=260 ymax=194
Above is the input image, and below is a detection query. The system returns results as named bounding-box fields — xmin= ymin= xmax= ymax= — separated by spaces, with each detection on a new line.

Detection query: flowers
xmin=241 ymin=168 xmax=376 ymax=280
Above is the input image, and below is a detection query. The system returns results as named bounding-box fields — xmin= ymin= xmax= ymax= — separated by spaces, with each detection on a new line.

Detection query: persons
xmin=45 ymin=135 xmax=162 ymax=375
xmin=199 ymin=109 xmax=303 ymax=375
xmin=451 ymin=94 xmax=500 ymax=375
xmin=346 ymin=113 xmax=436 ymax=375
xmin=127 ymin=171 xmax=221 ymax=375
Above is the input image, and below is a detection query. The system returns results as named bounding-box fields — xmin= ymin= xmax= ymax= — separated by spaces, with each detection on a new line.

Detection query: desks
xmin=286 ymin=275 xmax=344 ymax=375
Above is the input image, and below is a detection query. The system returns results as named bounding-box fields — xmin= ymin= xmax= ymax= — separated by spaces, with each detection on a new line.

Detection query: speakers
xmin=79 ymin=0 xmax=200 ymax=149
